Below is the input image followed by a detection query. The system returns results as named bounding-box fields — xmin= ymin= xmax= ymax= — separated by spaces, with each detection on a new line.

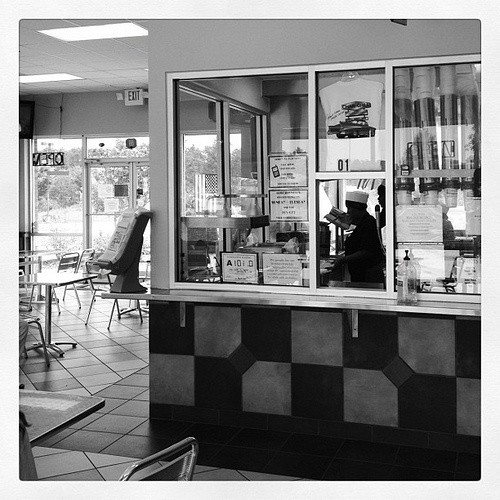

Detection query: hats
xmin=345 ymin=191 xmax=369 ymax=203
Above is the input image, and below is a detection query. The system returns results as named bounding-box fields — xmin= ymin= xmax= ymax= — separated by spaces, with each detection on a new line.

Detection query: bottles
xmin=396 ymin=250 xmax=418 ymax=307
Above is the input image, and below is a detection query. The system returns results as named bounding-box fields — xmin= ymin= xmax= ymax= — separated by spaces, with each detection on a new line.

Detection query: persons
xmin=333 ymin=192 xmax=386 ymax=282
xmin=378 ymin=180 xmax=386 ymax=228
xmin=442 ymin=204 xmax=457 ymax=249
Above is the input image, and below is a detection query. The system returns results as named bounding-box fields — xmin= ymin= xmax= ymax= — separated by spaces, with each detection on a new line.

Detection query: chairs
xmin=18 ymin=249 xmax=150 ymax=370
xmin=117 ymin=437 xmax=198 ymax=482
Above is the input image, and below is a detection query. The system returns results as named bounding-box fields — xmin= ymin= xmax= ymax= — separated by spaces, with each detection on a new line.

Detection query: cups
xmin=395 ymin=66 xmax=476 ymax=100
xmin=394 ymin=188 xmax=476 ymax=213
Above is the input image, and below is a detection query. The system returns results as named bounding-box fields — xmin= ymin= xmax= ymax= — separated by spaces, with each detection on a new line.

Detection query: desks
xmin=19 ymin=388 xmax=105 ymax=448
xmin=18 ymin=271 xmax=98 ymax=356
